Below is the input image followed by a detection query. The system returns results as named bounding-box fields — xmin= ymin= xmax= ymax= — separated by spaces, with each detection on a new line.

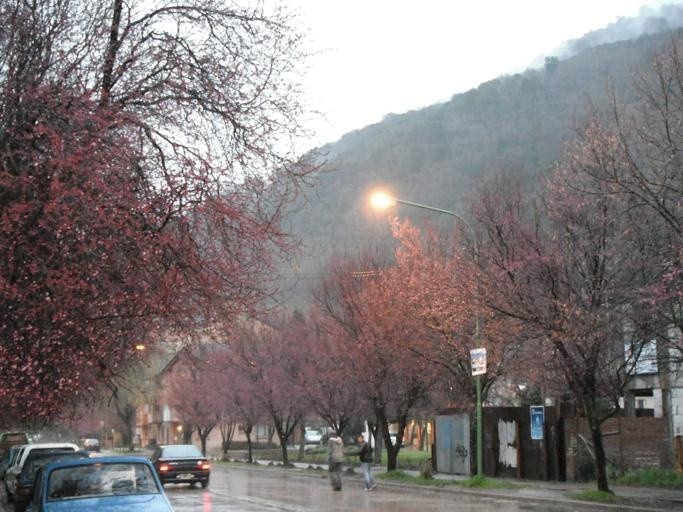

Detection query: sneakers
xmin=334 ymin=487 xmax=342 ymax=491
xmin=364 ymin=483 xmax=377 ymax=491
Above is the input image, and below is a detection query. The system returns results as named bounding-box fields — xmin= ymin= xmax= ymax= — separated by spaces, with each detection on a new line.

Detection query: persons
xmin=327 ymin=429 xmax=378 ymax=492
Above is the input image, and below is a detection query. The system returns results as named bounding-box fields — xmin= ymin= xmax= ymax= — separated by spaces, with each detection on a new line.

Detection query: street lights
xmin=371 ymin=190 xmax=483 ymax=475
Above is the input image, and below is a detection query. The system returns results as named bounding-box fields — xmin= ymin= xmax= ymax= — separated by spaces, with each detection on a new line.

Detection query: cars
xmin=305 ymin=426 xmax=404 ymax=448
xmin=1 ymin=431 xmax=209 ymax=512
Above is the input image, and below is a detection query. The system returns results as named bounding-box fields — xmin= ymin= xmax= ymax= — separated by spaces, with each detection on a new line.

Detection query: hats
xmin=329 ymin=430 xmax=336 ymax=436
